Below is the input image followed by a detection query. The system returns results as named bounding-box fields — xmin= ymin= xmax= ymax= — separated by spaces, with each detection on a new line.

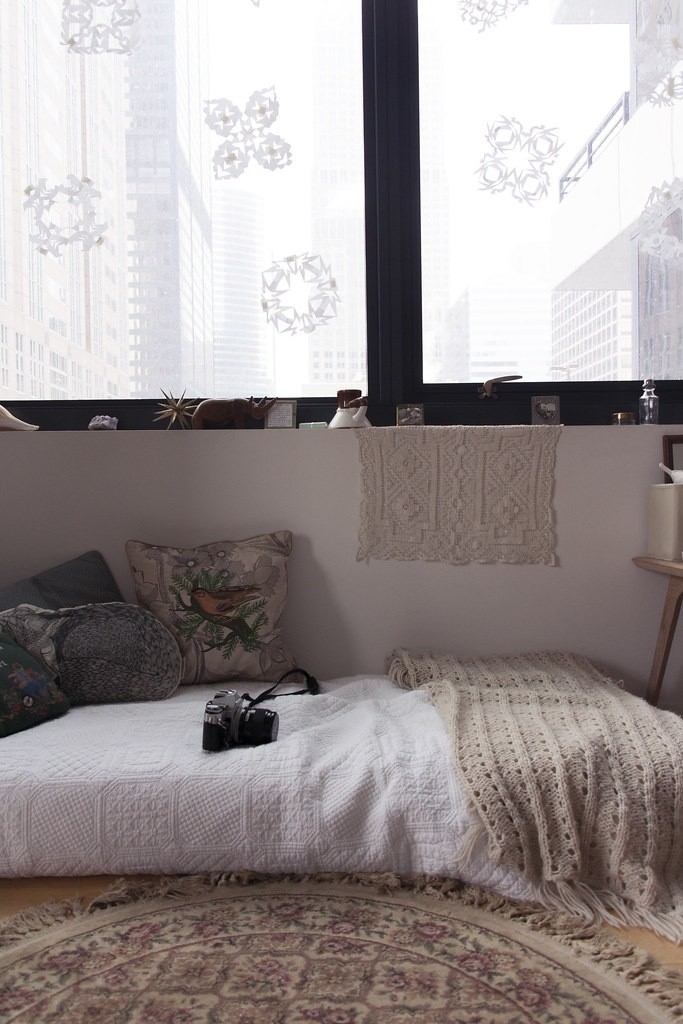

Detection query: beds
xmin=0 ymin=649 xmax=683 ymax=943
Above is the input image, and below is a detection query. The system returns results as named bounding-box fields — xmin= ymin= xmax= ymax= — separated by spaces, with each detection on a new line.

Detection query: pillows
xmin=125 ymin=531 xmax=303 ymax=687
xmin=0 ymin=630 xmax=70 ymax=738
xmin=0 ymin=550 xmax=124 ymax=613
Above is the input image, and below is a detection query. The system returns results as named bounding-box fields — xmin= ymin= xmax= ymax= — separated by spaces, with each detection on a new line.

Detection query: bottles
xmin=639 ymin=380 xmax=659 ymax=425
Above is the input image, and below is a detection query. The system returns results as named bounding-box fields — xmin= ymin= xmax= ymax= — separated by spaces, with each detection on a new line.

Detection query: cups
xmin=612 ymin=412 xmax=636 ymax=425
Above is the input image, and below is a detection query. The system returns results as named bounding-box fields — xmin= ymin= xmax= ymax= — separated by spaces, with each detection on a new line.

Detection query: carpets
xmin=0 ymin=870 xmax=683 ymax=1024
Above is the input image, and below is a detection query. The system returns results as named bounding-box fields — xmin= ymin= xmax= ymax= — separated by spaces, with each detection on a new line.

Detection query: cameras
xmin=202 ymin=689 xmax=279 ymax=754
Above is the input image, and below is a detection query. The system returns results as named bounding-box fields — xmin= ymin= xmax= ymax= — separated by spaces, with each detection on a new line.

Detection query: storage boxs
xmin=646 ymin=483 xmax=683 ymax=561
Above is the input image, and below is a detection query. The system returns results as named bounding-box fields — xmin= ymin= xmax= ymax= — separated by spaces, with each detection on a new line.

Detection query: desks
xmin=632 ymin=556 xmax=683 ymax=705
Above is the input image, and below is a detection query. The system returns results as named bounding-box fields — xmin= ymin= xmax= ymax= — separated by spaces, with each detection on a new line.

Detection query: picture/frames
xmin=663 ymin=434 xmax=683 ymax=484
